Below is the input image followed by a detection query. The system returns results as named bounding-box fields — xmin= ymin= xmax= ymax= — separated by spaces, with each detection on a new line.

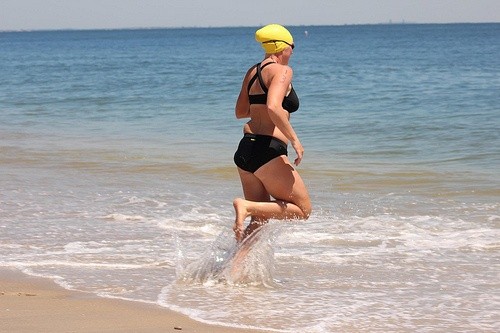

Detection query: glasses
xmin=263 ymin=40 xmax=294 ymax=50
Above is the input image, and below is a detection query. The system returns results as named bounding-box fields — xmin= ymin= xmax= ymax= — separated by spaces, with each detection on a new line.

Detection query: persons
xmin=230 ymin=22 xmax=313 ymax=243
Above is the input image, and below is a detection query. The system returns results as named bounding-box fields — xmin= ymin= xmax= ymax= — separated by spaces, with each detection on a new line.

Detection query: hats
xmin=255 ymin=24 xmax=294 ymax=55
